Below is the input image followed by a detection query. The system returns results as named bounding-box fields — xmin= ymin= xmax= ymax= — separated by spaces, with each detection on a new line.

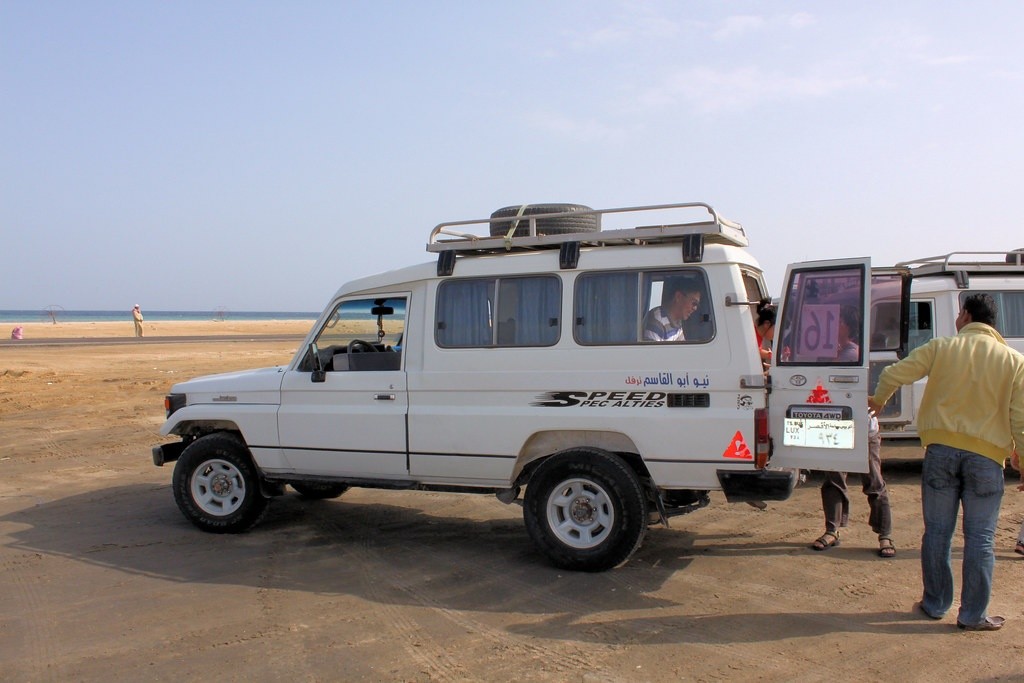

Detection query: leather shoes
xmin=918 ymin=600 xmax=944 ymax=620
xmin=957 ymin=616 xmax=1006 ymax=631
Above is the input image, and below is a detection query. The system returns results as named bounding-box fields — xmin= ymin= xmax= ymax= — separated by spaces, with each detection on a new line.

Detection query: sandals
xmin=878 ymin=536 xmax=897 ymax=556
xmin=813 ymin=530 xmax=840 ymax=550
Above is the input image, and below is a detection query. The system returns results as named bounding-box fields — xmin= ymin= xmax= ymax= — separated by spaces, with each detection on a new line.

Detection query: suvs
xmin=153 ymin=204 xmax=869 ymax=575
xmin=780 ymin=247 xmax=1023 ymax=458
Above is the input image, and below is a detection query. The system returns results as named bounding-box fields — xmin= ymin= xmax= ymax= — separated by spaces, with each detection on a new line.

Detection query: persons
xmin=814 ymin=308 xmax=896 ymax=558
xmin=754 ymin=294 xmax=791 ymax=440
xmin=133 ymin=304 xmax=144 ymax=338
xmin=867 ymin=293 xmax=1023 ymax=630
xmin=640 ymin=276 xmax=703 ymax=342
